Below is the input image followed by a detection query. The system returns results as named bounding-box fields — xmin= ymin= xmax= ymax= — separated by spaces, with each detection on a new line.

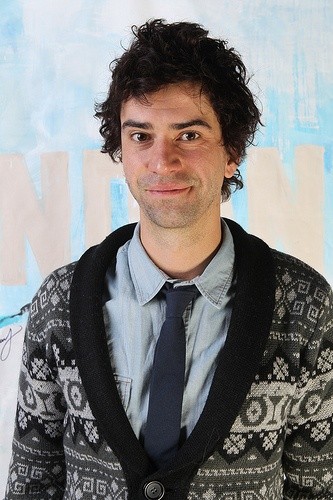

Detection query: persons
xmin=5 ymin=16 xmax=332 ymax=500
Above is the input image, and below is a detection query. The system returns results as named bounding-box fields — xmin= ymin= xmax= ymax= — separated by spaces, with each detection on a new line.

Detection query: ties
xmin=142 ymin=282 xmax=202 ymax=462
